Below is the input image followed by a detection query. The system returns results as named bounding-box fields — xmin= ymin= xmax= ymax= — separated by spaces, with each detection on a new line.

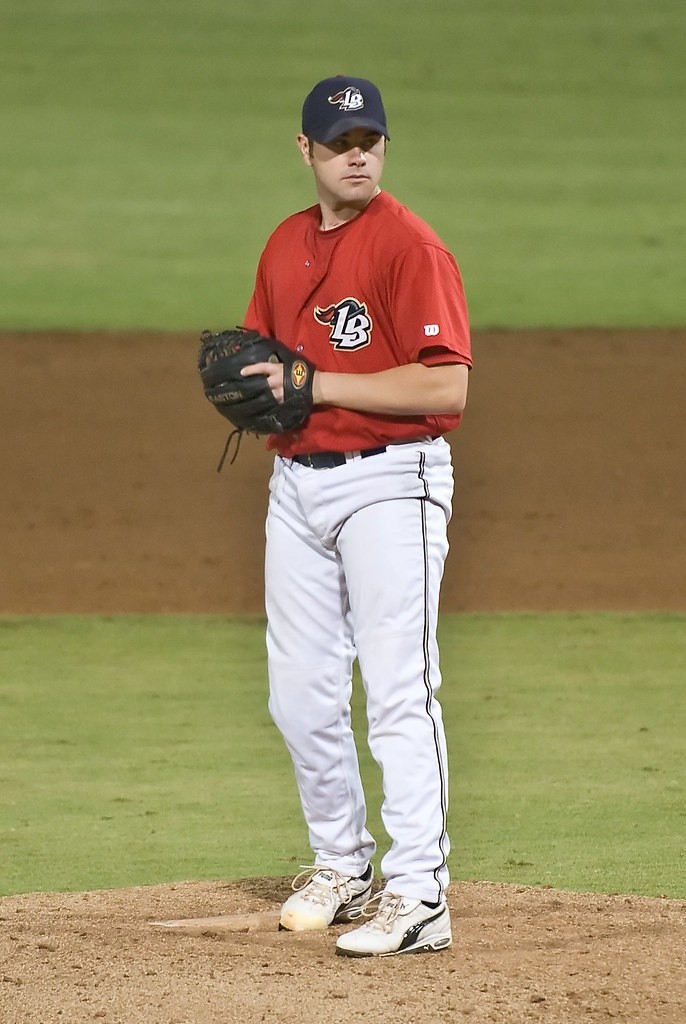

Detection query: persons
xmin=195 ymin=76 xmax=473 ymax=959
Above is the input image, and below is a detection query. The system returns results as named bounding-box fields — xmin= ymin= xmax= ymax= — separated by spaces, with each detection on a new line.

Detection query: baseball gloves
xmin=195 ymin=323 xmax=317 ymax=477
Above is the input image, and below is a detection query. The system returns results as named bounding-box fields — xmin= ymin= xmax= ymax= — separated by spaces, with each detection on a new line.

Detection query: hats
xmin=302 ymin=75 xmax=390 ymax=142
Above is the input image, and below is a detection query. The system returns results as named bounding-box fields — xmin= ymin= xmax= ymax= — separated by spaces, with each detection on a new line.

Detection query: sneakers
xmin=279 ymin=865 xmax=370 ymax=931
xmin=335 ymin=890 xmax=453 ymax=957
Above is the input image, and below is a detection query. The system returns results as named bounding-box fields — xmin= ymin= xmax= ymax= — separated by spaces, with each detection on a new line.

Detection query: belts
xmin=293 ymin=436 xmax=440 ymax=468
